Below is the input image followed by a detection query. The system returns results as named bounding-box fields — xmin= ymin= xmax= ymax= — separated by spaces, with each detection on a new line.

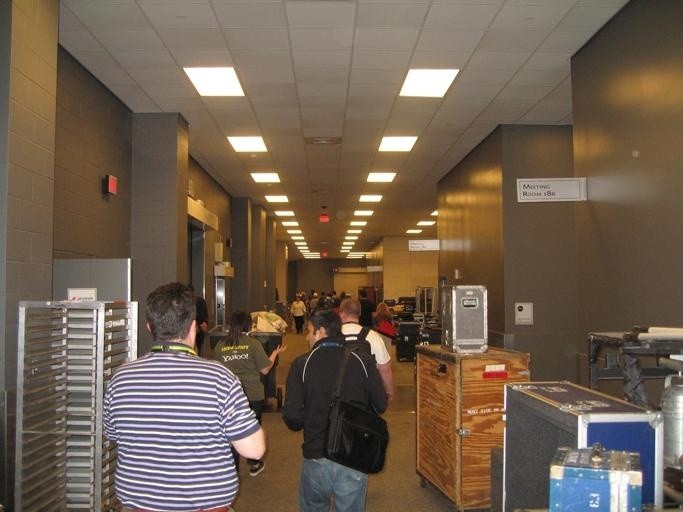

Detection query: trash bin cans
xmin=210 ymin=332 xmax=283 ymax=408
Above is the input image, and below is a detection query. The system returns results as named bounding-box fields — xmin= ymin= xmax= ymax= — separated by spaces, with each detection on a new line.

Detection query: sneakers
xmin=249 ymin=461 xmax=265 ymax=477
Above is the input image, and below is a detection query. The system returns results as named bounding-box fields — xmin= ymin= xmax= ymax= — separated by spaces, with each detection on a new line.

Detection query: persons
xmin=333 ymin=298 xmax=395 ymax=411
xmin=100 ymin=280 xmax=267 ymax=511
xmin=282 ymin=312 xmax=386 ymax=511
xmin=291 ymin=289 xmax=375 ymax=335
xmin=371 ymin=303 xmax=393 ymax=350
xmin=214 ymin=311 xmax=288 ymax=477
xmin=186 ymin=284 xmax=208 ymax=355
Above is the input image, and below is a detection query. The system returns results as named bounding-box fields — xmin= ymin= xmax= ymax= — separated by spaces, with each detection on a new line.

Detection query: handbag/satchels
xmin=378 ymin=319 xmax=396 ymax=337
xmin=325 ymin=401 xmax=388 ymax=474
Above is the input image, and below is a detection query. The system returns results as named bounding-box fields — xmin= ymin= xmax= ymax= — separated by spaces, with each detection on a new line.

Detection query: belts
xmin=131 ymin=506 xmax=229 ymax=512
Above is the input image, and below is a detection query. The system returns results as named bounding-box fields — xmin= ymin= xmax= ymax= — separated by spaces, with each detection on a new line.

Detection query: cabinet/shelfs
xmin=413 ymin=344 xmax=531 ymax=512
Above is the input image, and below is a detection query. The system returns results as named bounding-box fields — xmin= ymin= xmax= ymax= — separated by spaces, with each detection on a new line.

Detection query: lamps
xmin=319 ymin=205 xmax=329 ymax=225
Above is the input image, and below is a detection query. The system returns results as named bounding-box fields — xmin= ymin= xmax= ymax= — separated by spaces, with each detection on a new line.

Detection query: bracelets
xmin=385 ymin=392 xmax=392 ymax=402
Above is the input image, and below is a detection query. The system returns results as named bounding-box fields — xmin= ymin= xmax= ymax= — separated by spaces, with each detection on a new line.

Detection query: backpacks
xmin=339 ymin=325 xmax=371 ymax=354
